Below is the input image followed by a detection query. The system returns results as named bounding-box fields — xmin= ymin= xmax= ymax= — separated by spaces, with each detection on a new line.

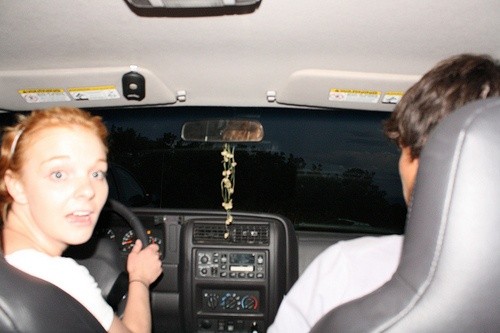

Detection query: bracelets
xmin=129 ymin=279 xmax=149 ymax=289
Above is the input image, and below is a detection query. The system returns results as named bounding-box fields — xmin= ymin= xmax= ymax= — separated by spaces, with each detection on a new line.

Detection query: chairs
xmin=310 ymin=94 xmax=500 ymax=332
xmin=1 ymin=249 xmax=111 ymax=333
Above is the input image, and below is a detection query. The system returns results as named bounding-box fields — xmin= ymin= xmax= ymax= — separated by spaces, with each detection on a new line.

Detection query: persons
xmin=0 ymin=106 xmax=164 ymax=333
xmin=265 ymin=54 xmax=500 ymax=333
